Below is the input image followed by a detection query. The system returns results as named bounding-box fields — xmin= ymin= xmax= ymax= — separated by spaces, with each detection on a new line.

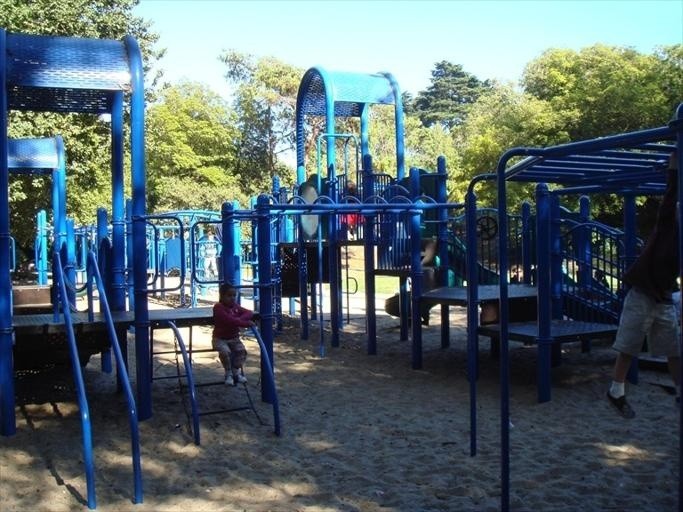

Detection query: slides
xmin=425 ymin=227 xmax=508 ymax=284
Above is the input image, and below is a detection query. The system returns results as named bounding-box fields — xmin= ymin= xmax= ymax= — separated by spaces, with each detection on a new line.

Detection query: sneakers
xmin=224 ymin=375 xmax=235 ymax=386
xmin=234 ymin=374 xmax=247 ymax=383
xmin=604 ymin=390 xmax=636 ymax=419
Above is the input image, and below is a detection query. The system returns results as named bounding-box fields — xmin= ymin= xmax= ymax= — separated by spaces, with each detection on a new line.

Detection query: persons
xmin=278 ymin=246 xmax=298 ymax=272
xmin=338 ymin=180 xmax=365 ymax=241
xmin=212 ymin=284 xmax=256 ymax=387
xmin=604 ymin=143 xmax=682 ymax=419
xmin=408 ymin=223 xmax=438 ymax=326
